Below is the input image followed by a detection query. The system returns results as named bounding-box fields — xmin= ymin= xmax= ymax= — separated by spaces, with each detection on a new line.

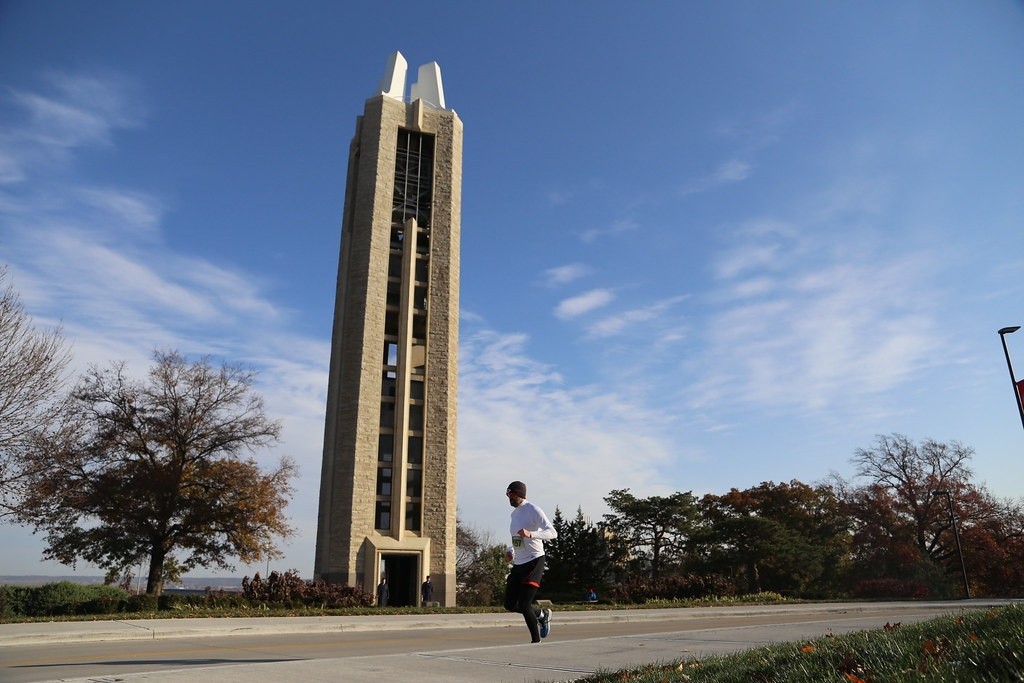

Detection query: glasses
xmin=506 ymin=491 xmax=511 ymax=497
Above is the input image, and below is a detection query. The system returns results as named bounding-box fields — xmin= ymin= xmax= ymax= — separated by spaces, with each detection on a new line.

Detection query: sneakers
xmin=537 ymin=609 xmax=552 ymax=638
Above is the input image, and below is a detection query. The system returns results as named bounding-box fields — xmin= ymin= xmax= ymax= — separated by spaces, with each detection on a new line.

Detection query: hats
xmin=508 ymin=481 xmax=526 ymax=499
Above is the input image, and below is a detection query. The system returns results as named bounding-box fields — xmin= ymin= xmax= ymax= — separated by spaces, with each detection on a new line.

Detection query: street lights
xmin=932 ymin=491 xmax=971 ymax=599
xmin=998 ymin=326 xmax=1024 ymax=428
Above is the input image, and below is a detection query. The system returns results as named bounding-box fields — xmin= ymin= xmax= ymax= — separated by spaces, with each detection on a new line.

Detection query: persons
xmin=422 ymin=575 xmax=433 ymax=602
xmin=377 ymin=578 xmax=389 ymax=607
xmin=504 ymin=482 xmax=558 ymax=643
xmin=586 ymin=588 xmax=596 ymax=601
